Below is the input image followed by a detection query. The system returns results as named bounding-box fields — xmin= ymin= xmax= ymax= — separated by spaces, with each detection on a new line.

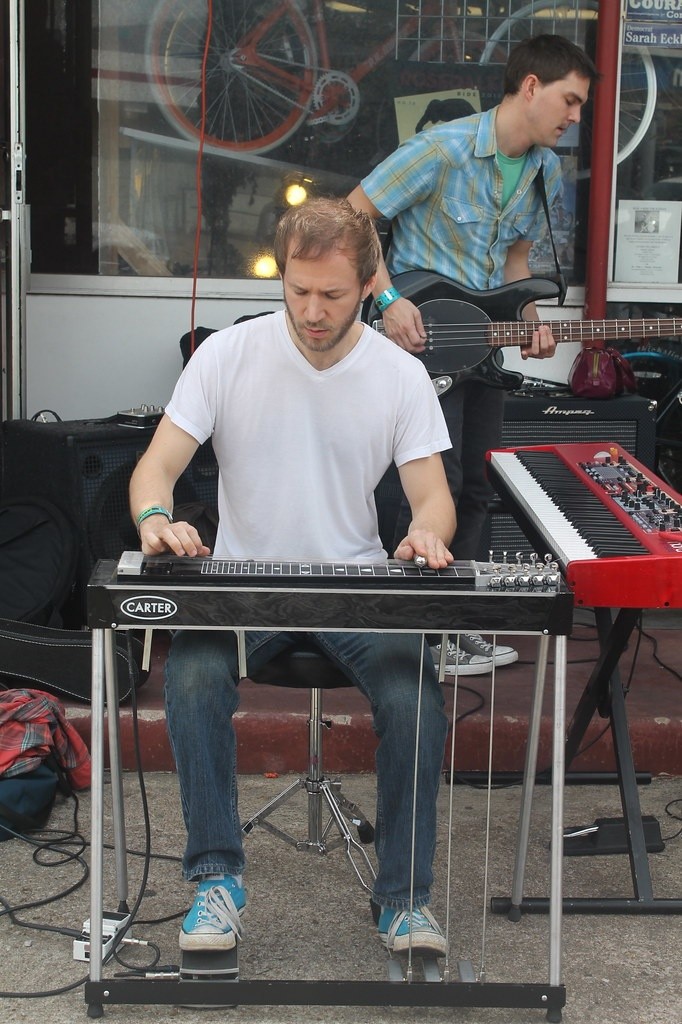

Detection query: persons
xmin=338 ymin=31 xmax=603 ymax=677
xmin=126 ymin=194 xmax=459 ymax=955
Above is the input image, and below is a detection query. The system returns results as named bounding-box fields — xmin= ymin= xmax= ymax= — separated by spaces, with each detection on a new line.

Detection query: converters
xmin=73 ymin=910 xmax=134 ymax=965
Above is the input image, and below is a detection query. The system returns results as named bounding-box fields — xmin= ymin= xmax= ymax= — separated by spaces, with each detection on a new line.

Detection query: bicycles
xmin=143 ymin=2 xmax=659 ymax=182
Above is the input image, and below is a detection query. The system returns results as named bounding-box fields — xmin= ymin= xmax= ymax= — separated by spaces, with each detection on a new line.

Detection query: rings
xmin=401 ymin=348 xmax=405 ymax=350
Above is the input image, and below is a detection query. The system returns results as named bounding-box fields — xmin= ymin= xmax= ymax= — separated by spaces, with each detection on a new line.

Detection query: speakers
xmin=493 ymin=393 xmax=658 ymax=564
xmin=0 ymin=417 xmax=219 ymax=630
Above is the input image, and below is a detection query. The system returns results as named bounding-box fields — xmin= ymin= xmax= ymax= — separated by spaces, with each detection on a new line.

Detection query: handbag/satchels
xmin=567 ymin=345 xmax=637 ymax=399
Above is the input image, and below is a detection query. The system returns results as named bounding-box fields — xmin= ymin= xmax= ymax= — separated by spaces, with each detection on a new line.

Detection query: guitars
xmin=362 ymin=269 xmax=682 ymax=402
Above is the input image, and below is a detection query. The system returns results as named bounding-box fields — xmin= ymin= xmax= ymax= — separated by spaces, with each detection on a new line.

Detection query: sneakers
xmin=424 ymin=637 xmax=494 ymax=676
xmin=377 ymin=905 xmax=449 ymax=959
xmin=179 ymin=873 xmax=249 ymax=952
xmin=448 ymin=633 xmax=519 ymax=668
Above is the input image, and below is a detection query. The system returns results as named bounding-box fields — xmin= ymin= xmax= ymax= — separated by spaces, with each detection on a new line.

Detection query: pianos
xmin=447 ymin=442 xmax=682 ymax=914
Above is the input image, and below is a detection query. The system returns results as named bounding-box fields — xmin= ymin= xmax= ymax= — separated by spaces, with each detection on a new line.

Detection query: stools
xmin=238 ymin=650 xmax=383 ymax=923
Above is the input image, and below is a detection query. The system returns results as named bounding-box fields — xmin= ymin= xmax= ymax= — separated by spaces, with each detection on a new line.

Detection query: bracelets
xmin=136 ymin=505 xmax=173 ymax=540
xmin=373 ymin=286 xmax=401 ymax=313
